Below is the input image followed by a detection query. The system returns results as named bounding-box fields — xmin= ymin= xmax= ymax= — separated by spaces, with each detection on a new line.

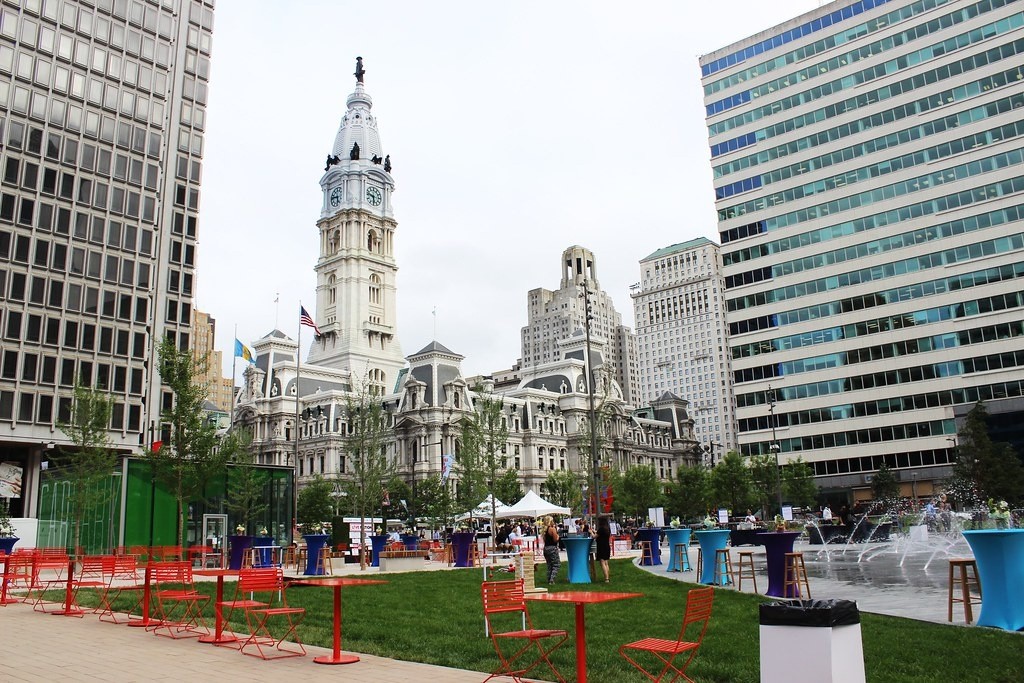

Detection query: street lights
xmin=410 ymin=457 xmax=430 ymax=533
xmin=420 ymin=438 xmax=447 ymax=514
xmin=766 ymin=383 xmax=783 ymax=515
xmin=577 ymin=278 xmax=601 ymax=530
xmin=704 ymin=440 xmax=724 ymax=468
xmin=946 ymin=438 xmax=959 ymax=464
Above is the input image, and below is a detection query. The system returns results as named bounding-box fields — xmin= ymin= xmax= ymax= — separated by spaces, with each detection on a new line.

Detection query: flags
xmin=301 ymin=305 xmax=320 ymax=336
xmin=234 ymin=338 xmax=256 ymax=363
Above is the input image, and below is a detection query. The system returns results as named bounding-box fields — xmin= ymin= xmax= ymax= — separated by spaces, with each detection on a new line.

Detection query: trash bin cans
xmin=759 ymin=599 xmax=866 ymax=683
xmin=908 ymin=524 xmax=928 ymax=541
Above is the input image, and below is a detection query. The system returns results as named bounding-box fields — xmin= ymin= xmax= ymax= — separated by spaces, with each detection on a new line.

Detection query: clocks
xmin=366 ymin=186 xmax=382 ymax=206
xmin=330 ymin=187 xmax=342 ymax=206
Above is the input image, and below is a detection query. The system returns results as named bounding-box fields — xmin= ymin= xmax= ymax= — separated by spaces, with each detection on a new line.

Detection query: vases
xmin=408 ymin=534 xmax=412 ymax=536
xmin=237 ymin=531 xmax=243 ymax=536
xmin=261 ymin=534 xmax=265 ymax=538
xmin=376 ymin=532 xmax=380 ymax=536
xmin=648 ymin=526 xmax=652 ymax=529
xmin=776 ymin=526 xmax=785 ymax=533
xmin=707 ymin=525 xmax=714 ymax=531
xmin=996 ymin=518 xmax=1011 ymax=530
xmin=673 ymin=525 xmax=678 ymax=529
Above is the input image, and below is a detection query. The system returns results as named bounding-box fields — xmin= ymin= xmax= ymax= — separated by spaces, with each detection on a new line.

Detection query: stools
xmin=240 ymin=542 xmax=481 ymax=575
xmin=736 ymin=551 xmax=758 ymax=594
xmin=715 ymin=548 xmax=736 ymax=587
xmin=673 ymin=543 xmax=692 ymax=573
xmin=947 ymin=558 xmax=984 ymax=624
xmin=783 ymin=553 xmax=812 ymax=600
xmin=640 ymin=541 xmax=654 ymax=567
xmin=696 ymin=548 xmax=703 ymax=583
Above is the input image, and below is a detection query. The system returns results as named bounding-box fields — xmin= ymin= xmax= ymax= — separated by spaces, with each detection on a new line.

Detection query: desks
xmin=756 ymin=531 xmax=804 ymax=596
xmin=0 ymin=538 xmax=20 ymax=583
xmin=0 ymin=556 xmax=34 ymax=603
xmin=562 ymin=537 xmax=592 ymax=584
xmin=512 ymin=590 xmax=647 ymax=683
xmin=49 ymin=558 xmax=90 ymax=616
xmin=664 ymin=529 xmax=693 ymax=571
xmin=961 ymin=529 xmax=1024 ymax=631
xmin=189 ymin=569 xmax=243 ymax=645
xmin=294 ymin=579 xmax=388 ymax=664
xmin=404 ymin=536 xmax=419 ymax=551
xmin=300 ymin=534 xmax=329 ymax=575
xmin=695 ymin=530 xmax=732 ymax=586
xmin=639 ymin=528 xmax=663 ymax=565
xmin=399 ymin=533 xmax=407 ymax=545
xmin=125 ymin=565 xmax=172 ymax=627
xmin=252 ymin=537 xmax=274 ymax=566
xmin=228 ymin=535 xmax=255 ymax=570
xmin=369 ymin=535 xmax=389 ymax=567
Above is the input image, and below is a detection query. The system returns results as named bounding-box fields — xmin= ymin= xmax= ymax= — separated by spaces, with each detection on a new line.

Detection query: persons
xmin=388 ymin=527 xmax=442 ymax=548
xmin=541 ymin=516 xmax=561 ymax=584
xmin=487 ymin=519 xmax=532 ymax=559
xmin=588 ymin=516 xmax=611 ymax=583
xmin=609 ymin=517 xmax=645 ymax=550
xmin=796 ymin=499 xmax=869 ymax=544
xmin=558 ymin=519 xmax=596 ymax=551
xmin=925 ymin=493 xmax=1010 ymax=532
xmin=670 ymin=510 xmax=763 ymax=530
xmin=350 ymin=142 xmax=360 ymax=160
xmin=385 ymin=154 xmax=392 ymax=173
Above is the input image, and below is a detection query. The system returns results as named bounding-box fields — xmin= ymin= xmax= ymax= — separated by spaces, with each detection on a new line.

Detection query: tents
xmin=455 ymin=490 xmax=572 ymax=560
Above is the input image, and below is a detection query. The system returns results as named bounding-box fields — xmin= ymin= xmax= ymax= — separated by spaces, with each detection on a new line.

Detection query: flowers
xmin=376 ymin=526 xmax=383 ymax=533
xmin=236 ymin=524 xmax=244 ymax=531
xmin=295 ymin=521 xmax=333 ymax=540
xmin=260 ymin=527 xmax=268 ymax=535
xmin=704 ymin=516 xmax=717 ymax=527
xmin=987 ymin=499 xmax=1010 ymax=521
xmin=406 ymin=529 xmax=413 ymax=534
xmin=672 ymin=519 xmax=680 ymax=526
xmin=646 ymin=520 xmax=654 ymax=526
xmin=774 ymin=514 xmax=786 ymax=527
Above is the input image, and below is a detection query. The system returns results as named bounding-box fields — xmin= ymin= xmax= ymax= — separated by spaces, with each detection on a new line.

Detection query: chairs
xmin=0 ymin=545 xmax=307 ymax=660
xmin=482 ymin=581 xmax=568 ymax=683
xmin=620 ymin=586 xmax=714 ymax=683
